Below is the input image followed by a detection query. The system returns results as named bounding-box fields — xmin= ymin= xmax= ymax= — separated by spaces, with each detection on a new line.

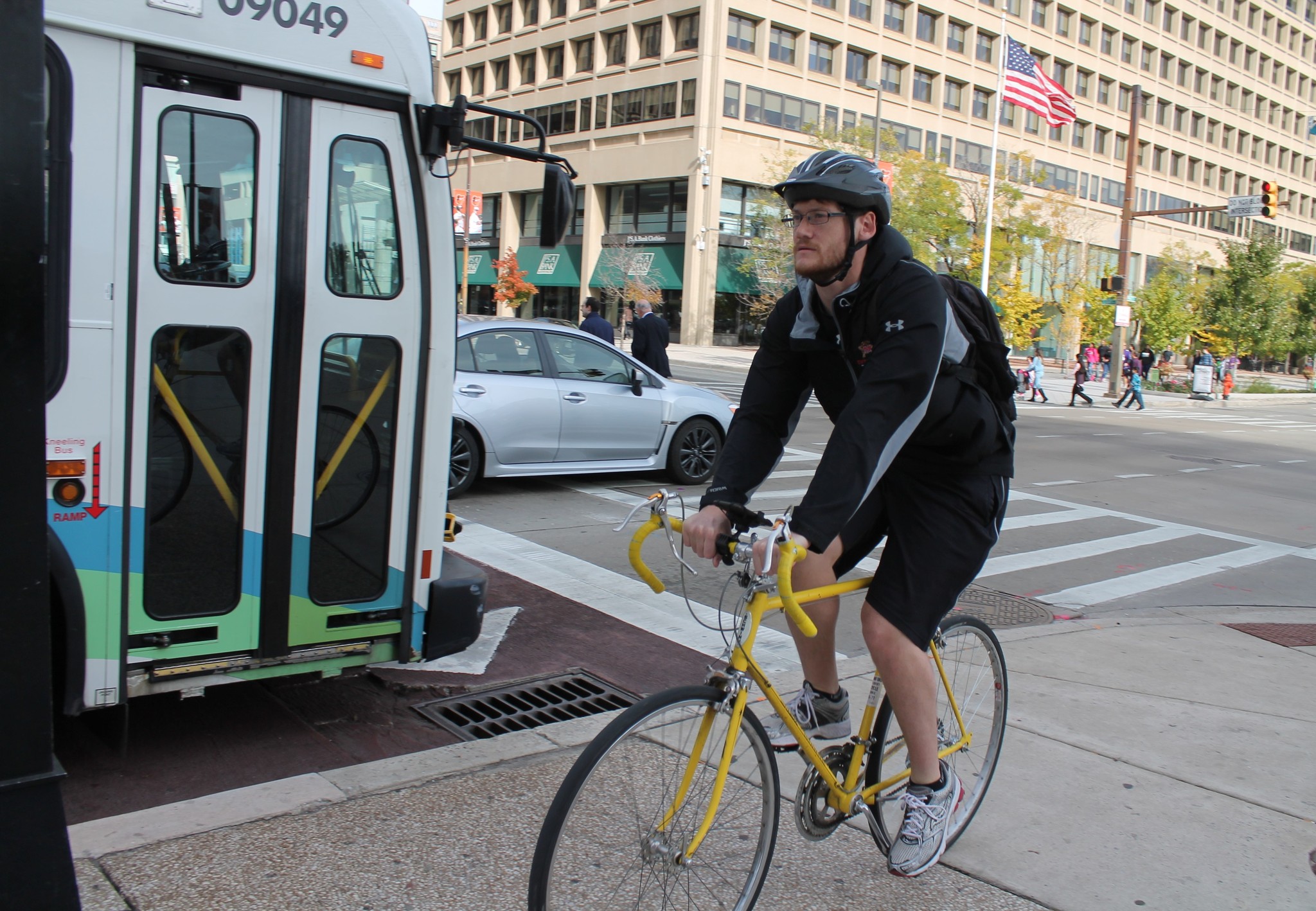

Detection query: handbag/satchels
xmin=1083 ymin=367 xmax=1090 ymax=382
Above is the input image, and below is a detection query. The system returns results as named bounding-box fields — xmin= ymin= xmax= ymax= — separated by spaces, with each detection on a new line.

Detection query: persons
xmin=1111 ymin=351 xmax=1144 ymax=410
xmin=624 ymin=304 xmax=633 ymax=340
xmin=453 ymin=205 xmax=465 ymax=232
xmin=1022 ymin=347 xmax=1048 ymax=403
xmin=573 ymin=297 xmax=614 ymax=368
xmin=1124 ymin=342 xmax=1154 ymax=380
xmin=1084 ymin=339 xmax=1113 ymax=382
xmin=1159 ymin=344 xmax=1174 ymax=382
xmin=631 ymin=299 xmax=672 ymax=378
xmin=1064 ymin=353 xmax=1092 ymax=407
xmin=469 ymin=207 xmax=482 ymax=234
xmin=1192 ymin=347 xmax=1241 ymax=382
xmin=682 ymin=152 xmax=1017 ymax=878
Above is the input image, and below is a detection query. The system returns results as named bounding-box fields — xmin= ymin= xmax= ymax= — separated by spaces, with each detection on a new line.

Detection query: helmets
xmin=774 ymin=148 xmax=891 ymax=225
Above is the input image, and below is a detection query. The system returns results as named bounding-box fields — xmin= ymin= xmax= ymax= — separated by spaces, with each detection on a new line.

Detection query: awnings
xmin=456 ymin=247 xmax=497 ymax=285
xmin=514 ymin=245 xmax=582 ymax=287
xmin=716 ymin=246 xmax=797 ymax=296
xmin=589 ymin=244 xmax=685 ymax=289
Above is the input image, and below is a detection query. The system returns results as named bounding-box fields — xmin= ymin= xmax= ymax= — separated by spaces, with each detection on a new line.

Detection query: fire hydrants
xmin=1221 ymin=372 xmax=1235 ymax=400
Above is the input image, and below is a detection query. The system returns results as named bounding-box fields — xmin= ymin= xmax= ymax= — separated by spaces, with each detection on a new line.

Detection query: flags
xmin=1000 ymin=35 xmax=1077 ymax=128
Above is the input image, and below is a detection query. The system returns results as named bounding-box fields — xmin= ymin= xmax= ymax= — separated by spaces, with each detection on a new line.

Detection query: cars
xmin=332 ymin=315 xmax=737 ymax=500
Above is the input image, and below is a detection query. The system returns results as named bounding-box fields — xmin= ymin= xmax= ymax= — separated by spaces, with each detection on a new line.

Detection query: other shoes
xmin=1112 ymin=402 xmax=1119 ymax=408
xmin=1124 ymin=405 xmax=1129 ymax=410
xmin=1028 ymin=398 xmax=1034 ymax=402
xmin=1088 ymin=399 xmax=1094 ymax=407
xmin=1067 ymin=403 xmax=1074 ymax=407
xmin=1041 ymin=398 xmax=1047 ymax=403
xmin=1093 ymin=377 xmax=1097 ymax=382
xmin=1137 ymin=408 xmax=1145 ymax=411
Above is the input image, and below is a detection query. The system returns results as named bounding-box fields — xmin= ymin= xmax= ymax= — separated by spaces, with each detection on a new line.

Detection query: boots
xmin=1091 ymin=375 xmax=1095 ymax=381
xmin=1098 ymin=377 xmax=1103 ymax=382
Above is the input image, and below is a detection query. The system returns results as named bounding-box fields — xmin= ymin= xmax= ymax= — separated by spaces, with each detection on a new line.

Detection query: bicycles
xmin=526 ymin=487 xmax=1011 ymax=910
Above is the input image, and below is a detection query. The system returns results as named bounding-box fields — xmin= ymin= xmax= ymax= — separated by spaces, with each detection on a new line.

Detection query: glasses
xmin=778 ymin=207 xmax=848 ymax=226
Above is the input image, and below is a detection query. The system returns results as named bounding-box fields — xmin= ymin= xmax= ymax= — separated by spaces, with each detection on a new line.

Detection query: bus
xmin=19 ymin=1 xmax=581 ymax=744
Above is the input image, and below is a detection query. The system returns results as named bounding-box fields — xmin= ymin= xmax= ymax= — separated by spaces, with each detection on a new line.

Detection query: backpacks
xmin=863 ymin=265 xmax=1019 ymax=464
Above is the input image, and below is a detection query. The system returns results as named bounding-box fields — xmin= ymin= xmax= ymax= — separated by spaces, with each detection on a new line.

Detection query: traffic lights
xmin=1105 ymin=276 xmax=1124 ymax=291
xmin=1260 ymin=180 xmax=1279 ymax=218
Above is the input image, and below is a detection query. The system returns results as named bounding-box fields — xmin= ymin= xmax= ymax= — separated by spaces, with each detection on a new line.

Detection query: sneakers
xmin=755 ymin=679 xmax=851 ymax=749
xmin=885 ymin=755 xmax=964 ymax=879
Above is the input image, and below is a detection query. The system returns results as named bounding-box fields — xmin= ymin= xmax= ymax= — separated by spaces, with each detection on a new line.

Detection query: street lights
xmin=856 ymin=77 xmax=883 ymax=169
